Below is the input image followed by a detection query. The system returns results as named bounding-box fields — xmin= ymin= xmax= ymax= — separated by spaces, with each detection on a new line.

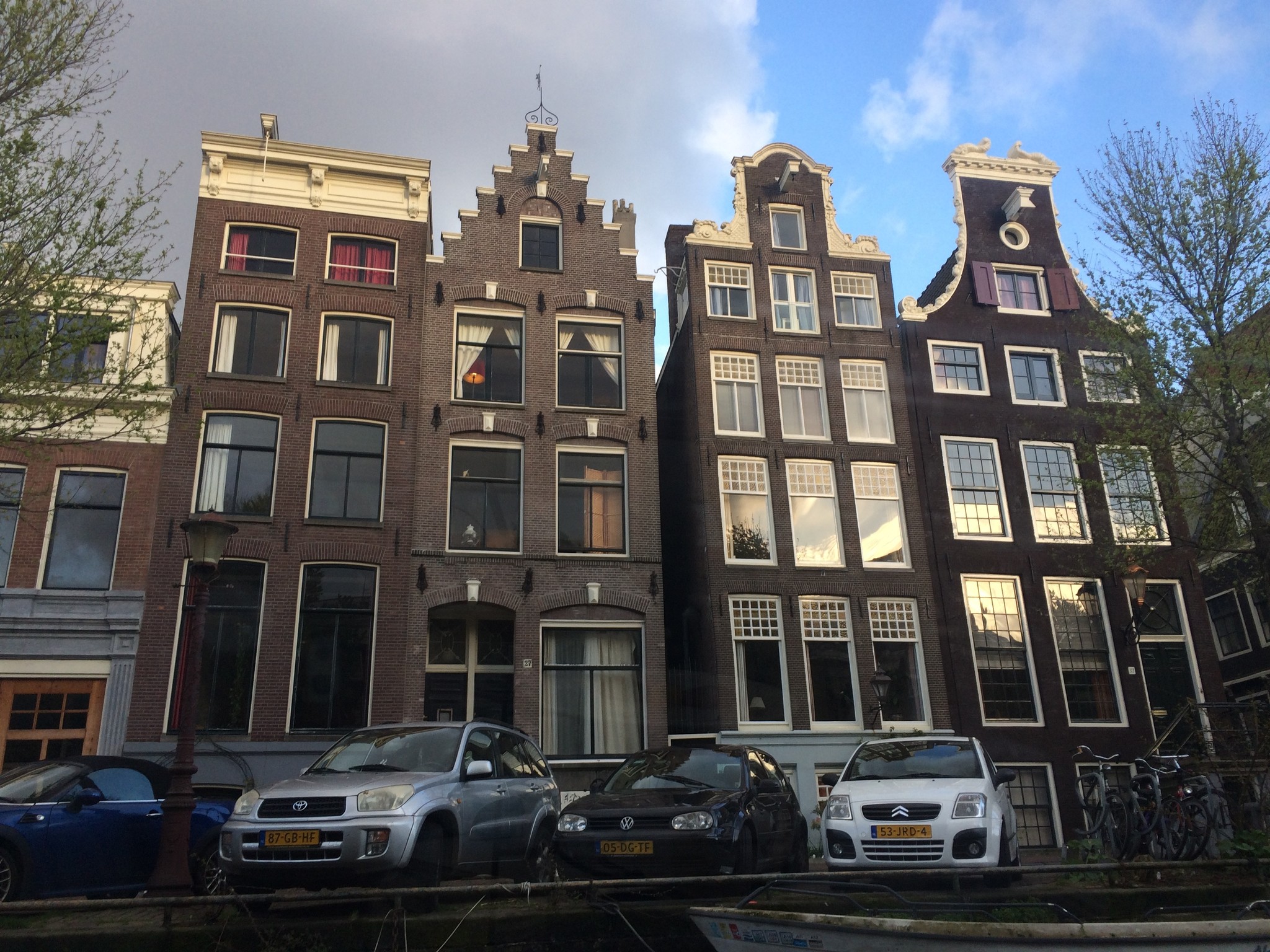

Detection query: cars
xmin=551 ymin=744 xmax=811 ymax=900
xmin=0 ymin=757 xmax=242 ymax=897
xmin=819 ymin=736 xmax=1025 ymax=889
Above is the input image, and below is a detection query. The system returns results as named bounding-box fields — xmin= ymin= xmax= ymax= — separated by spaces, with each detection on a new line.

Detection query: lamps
xmin=1118 ymin=555 xmax=1149 ymax=647
xmin=869 ymin=661 xmax=893 ymax=728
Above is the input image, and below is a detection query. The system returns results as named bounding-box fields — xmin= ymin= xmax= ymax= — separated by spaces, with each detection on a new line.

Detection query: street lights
xmin=152 ymin=507 xmax=240 ymax=907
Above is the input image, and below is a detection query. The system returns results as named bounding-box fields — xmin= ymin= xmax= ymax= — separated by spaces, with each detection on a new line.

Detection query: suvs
xmin=218 ymin=719 xmax=564 ymax=914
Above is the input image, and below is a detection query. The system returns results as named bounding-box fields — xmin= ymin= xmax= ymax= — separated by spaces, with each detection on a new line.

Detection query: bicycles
xmin=1071 ymin=747 xmax=1214 ymax=862
